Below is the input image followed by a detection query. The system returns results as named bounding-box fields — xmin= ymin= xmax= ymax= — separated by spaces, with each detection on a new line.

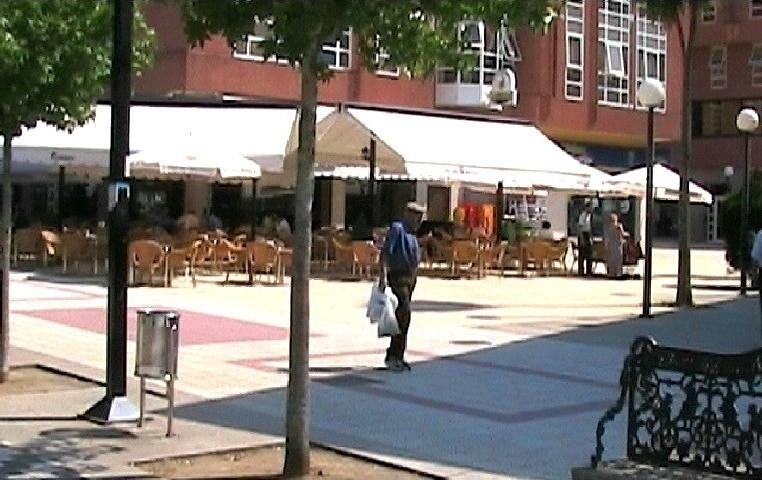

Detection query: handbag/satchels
xmin=366 ymin=283 xmax=400 ymax=337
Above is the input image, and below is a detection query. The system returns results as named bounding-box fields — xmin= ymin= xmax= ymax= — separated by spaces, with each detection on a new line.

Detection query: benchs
xmin=567 ymin=336 xmax=762 ymax=480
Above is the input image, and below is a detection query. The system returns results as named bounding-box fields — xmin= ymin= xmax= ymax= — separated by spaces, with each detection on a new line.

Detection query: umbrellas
xmin=119 ymin=143 xmax=264 ymax=230
xmin=605 ymin=154 xmax=718 ymax=212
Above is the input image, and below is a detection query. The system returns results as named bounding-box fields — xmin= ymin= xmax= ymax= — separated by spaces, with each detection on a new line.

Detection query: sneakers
xmin=385 ymin=348 xmax=411 ymax=373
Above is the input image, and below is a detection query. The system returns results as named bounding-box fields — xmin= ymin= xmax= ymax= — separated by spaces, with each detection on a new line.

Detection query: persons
xmin=13 ymin=185 xmax=294 ymax=238
xmin=602 ymin=212 xmax=626 ymax=278
xmin=575 ymin=201 xmax=596 ymax=277
xmin=538 ymin=219 xmax=554 ymax=242
xmin=378 ymin=201 xmax=427 ymax=371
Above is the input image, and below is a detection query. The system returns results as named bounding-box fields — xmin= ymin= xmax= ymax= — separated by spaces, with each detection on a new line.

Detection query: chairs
xmin=14 ymin=219 xmax=641 ymax=288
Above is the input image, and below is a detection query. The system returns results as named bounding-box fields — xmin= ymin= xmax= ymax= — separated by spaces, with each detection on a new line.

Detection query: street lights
xmin=635 ymin=77 xmax=668 ymax=317
xmin=736 ymin=109 xmax=760 ymax=294
